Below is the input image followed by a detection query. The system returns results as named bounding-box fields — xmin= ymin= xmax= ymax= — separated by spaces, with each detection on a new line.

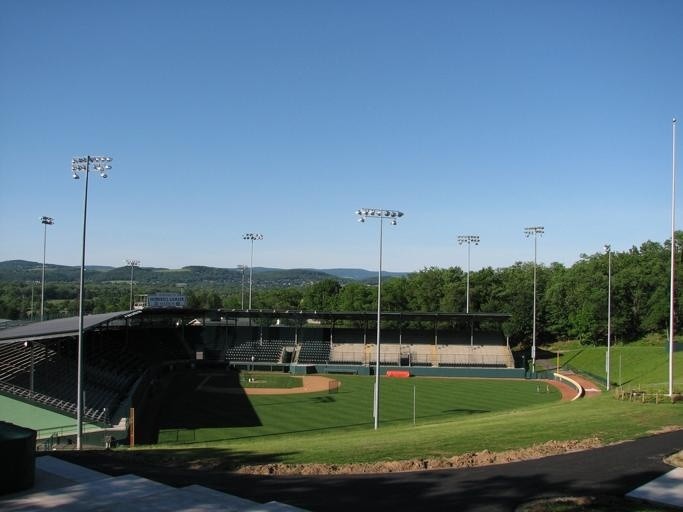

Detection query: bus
xmin=130 ymin=291 xmax=186 ymax=312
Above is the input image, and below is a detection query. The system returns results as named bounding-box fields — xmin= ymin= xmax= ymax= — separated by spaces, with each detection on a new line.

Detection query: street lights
xmin=354 ymin=207 xmax=404 ymax=429
xmin=240 ymin=230 xmax=264 ymax=310
xmin=602 ymin=244 xmax=611 ymax=392
xmin=523 ymin=224 xmax=544 ymax=374
xmin=122 ymin=256 xmax=141 ymax=311
xmin=38 ymin=215 xmax=55 ymax=322
xmin=456 ymin=231 xmax=481 ymax=313
xmin=236 ymin=263 xmax=249 ymax=310
xmin=24 ymin=278 xmax=39 ymax=325
xmin=67 ymin=154 xmax=114 ymax=451
